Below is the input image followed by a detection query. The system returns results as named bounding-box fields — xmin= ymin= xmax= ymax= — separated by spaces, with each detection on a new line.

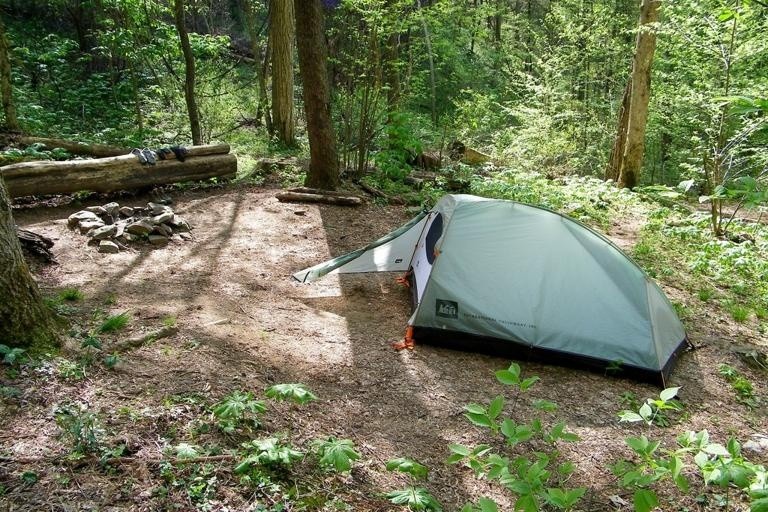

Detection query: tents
xmin=291 ymin=193 xmax=696 ymax=393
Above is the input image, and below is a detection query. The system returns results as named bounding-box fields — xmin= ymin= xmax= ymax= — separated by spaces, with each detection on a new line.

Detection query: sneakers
xmin=132 ymin=149 xmax=147 ymax=164
xmin=142 ymin=148 xmax=156 ymax=164
